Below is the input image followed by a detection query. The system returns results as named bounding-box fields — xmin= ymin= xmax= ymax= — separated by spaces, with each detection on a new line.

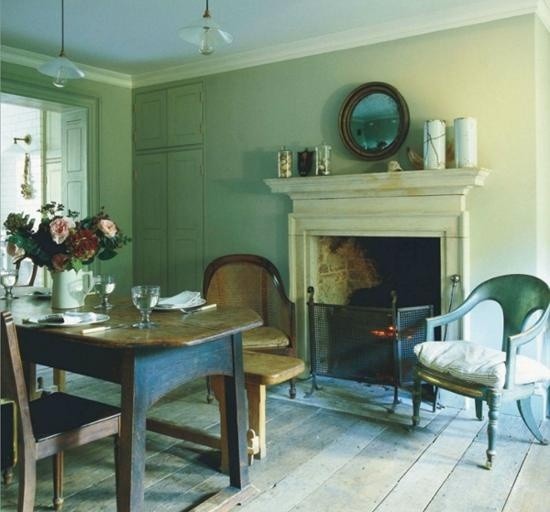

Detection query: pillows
xmin=414 ymin=341 xmax=548 ymax=389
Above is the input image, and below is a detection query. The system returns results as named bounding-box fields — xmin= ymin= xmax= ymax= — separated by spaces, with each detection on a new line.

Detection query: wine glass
xmin=0 ymin=270 xmax=19 ymax=300
xmin=94 ymin=275 xmax=116 ymax=310
xmin=130 ymin=284 xmax=161 ymax=328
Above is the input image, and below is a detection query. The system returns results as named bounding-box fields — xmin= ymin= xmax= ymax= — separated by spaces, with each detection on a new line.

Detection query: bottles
xmin=277 ymin=146 xmax=293 ymax=178
xmin=315 ymin=142 xmax=332 ymax=176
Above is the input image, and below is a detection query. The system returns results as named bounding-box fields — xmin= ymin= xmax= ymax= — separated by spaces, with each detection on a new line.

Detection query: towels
xmin=38 ymin=312 xmax=97 ymax=326
xmin=157 ymin=288 xmax=202 ymax=311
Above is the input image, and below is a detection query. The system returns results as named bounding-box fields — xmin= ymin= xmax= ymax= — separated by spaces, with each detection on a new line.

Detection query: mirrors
xmin=337 ymin=81 xmax=412 ymax=161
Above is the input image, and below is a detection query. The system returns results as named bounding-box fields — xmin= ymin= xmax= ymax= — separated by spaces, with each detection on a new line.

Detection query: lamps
xmin=3 ymin=133 xmax=32 ymax=155
xmin=39 ymin=2 xmax=90 ymax=90
xmin=180 ymin=1 xmax=233 ymax=56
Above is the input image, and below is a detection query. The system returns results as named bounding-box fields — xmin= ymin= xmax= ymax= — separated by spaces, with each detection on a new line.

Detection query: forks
xmin=179 ymin=303 xmax=217 ymax=313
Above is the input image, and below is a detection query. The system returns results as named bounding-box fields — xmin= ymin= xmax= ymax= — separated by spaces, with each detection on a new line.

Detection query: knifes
xmin=81 ymin=322 xmax=129 ymax=334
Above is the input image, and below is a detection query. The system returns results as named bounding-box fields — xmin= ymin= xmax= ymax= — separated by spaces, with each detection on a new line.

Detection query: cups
xmin=423 ymin=118 xmax=447 ymax=168
xmin=50 ymin=269 xmax=94 ymax=310
xmin=454 ymin=117 xmax=479 ymax=168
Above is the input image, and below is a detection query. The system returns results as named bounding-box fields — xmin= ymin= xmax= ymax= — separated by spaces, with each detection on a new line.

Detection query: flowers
xmin=4 ymin=198 xmax=131 ymax=277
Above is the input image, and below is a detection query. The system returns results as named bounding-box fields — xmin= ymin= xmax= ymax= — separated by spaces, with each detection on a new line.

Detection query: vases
xmin=50 ymin=264 xmax=97 ymax=310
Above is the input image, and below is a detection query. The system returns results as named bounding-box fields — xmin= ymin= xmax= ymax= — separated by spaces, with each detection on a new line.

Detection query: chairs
xmin=1 ymin=311 xmax=123 ymax=511
xmin=201 ymin=254 xmax=297 ymax=404
xmin=412 ymin=272 xmax=549 ymax=468
xmin=10 ymin=252 xmax=44 ymax=286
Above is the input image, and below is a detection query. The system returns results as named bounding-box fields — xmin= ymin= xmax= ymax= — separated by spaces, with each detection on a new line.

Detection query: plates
xmin=155 ymin=297 xmax=206 ymax=311
xmin=28 ymin=291 xmax=51 ymax=297
xmin=29 ymin=311 xmax=111 ymax=327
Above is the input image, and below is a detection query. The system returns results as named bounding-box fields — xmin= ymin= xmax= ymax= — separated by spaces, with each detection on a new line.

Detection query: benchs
xmin=51 ymin=338 xmax=304 ymax=457
